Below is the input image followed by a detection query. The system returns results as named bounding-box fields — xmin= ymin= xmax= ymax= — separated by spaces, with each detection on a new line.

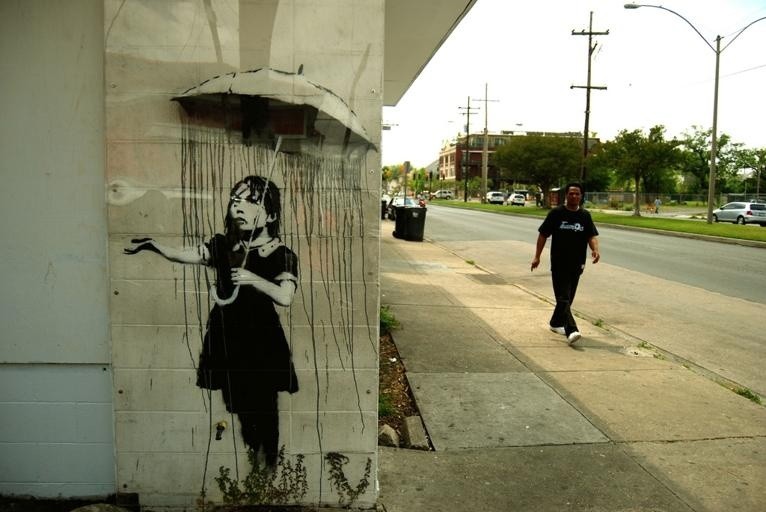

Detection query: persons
xmin=121 ymin=174 xmax=302 ymax=484
xmin=535 ymin=191 xmax=544 ymax=207
xmin=530 ymin=183 xmax=601 ymax=345
xmin=654 ymin=197 xmax=664 ymax=214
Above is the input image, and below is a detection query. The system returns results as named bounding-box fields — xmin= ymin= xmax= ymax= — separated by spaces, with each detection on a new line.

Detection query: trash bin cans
xmin=392 ymin=206 xmax=427 ymax=241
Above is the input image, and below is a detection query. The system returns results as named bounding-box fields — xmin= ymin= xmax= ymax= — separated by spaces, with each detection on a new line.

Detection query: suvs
xmin=712 ymin=201 xmax=765 ymax=228
xmin=507 ymin=193 xmax=525 ymax=207
xmin=431 ymin=190 xmax=452 ymax=200
xmin=484 ymin=191 xmax=504 ymax=206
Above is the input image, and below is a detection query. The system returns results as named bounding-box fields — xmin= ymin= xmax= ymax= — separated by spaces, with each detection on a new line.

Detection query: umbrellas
xmin=170 ymin=62 xmax=379 ymax=310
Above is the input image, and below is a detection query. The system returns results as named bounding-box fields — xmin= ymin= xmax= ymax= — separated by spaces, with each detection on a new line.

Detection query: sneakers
xmin=568 ymin=331 xmax=582 ymax=344
xmin=549 ymin=325 xmax=567 ymax=336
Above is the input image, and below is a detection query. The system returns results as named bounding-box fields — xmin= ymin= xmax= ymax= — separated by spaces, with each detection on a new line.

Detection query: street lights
xmin=623 ymin=4 xmax=766 ymax=224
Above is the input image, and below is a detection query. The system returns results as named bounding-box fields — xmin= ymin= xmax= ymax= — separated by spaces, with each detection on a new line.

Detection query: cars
xmin=382 ymin=191 xmax=420 ymax=220
